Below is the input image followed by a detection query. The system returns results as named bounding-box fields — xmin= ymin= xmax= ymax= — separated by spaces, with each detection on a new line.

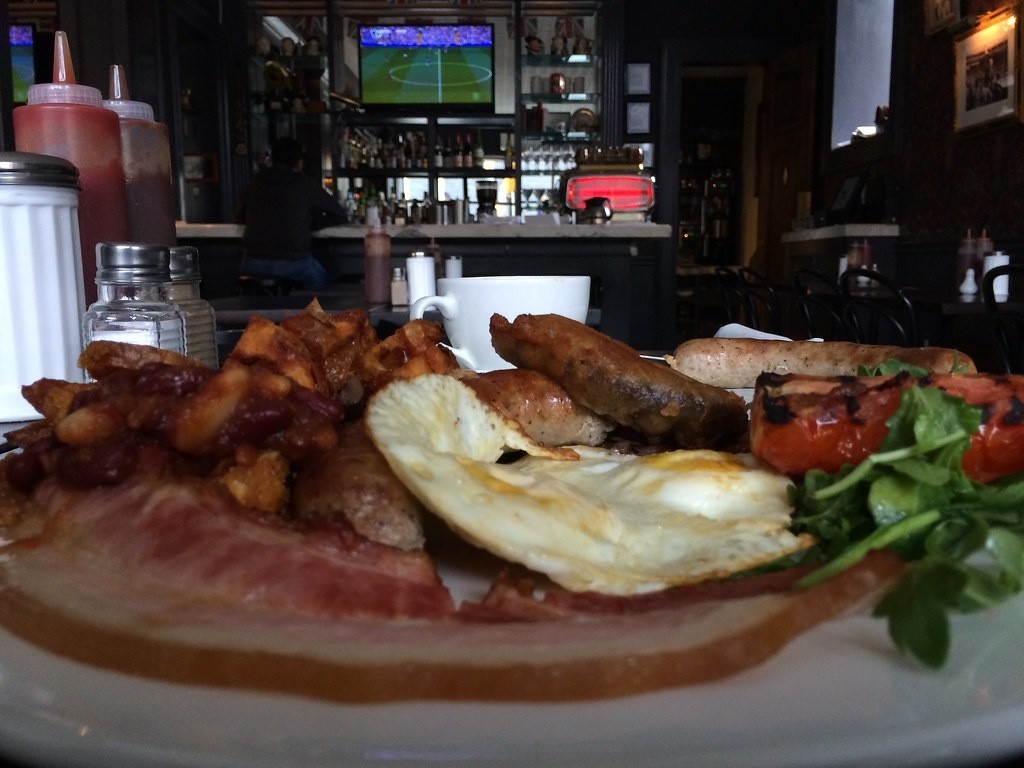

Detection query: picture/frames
xmin=923 ymin=0 xmax=961 ymax=38
xmin=951 ymin=9 xmax=1024 ymax=141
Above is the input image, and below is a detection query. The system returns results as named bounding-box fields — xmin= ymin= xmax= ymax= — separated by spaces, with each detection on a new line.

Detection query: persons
xmin=258 ymin=37 xmax=320 ymax=56
xmin=231 ymin=136 xmax=345 ymax=291
xmin=523 ymin=34 xmax=591 ymax=55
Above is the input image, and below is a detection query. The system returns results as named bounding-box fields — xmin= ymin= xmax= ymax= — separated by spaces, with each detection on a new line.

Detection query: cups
xmin=410 ymin=276 xmax=591 ymax=373
xmin=477 ymin=181 xmax=497 ymax=206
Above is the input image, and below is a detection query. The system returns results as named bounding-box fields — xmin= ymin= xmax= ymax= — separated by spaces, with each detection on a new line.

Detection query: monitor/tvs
xmin=9 ymin=22 xmax=38 ymax=105
xmin=358 ymin=23 xmax=495 ymax=115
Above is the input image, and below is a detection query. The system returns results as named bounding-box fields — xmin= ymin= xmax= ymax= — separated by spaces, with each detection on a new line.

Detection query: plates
xmin=572 ymin=109 xmax=598 ymax=131
xmin=0 ymin=389 xmax=1024 ymax=768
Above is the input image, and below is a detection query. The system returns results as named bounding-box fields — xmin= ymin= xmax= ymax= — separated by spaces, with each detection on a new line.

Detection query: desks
xmin=175 ymin=223 xmax=674 ymax=348
xmin=810 ymin=287 xmax=1024 ymax=354
xmin=755 ymin=282 xmax=848 ymax=342
xmin=208 ymin=295 xmax=601 ymax=347
xmin=780 ymin=223 xmax=900 ymax=336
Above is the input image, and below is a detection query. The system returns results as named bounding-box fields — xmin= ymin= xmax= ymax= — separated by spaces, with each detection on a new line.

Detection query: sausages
xmin=664 ymin=338 xmax=977 ymax=387
xmin=325 ymin=370 xmax=613 ymax=549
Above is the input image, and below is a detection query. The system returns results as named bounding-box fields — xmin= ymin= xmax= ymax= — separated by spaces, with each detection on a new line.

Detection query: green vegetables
xmin=717 ymin=349 xmax=1024 ymax=670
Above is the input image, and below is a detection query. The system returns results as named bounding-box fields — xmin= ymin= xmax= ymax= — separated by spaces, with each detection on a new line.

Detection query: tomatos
xmin=748 ymin=371 xmax=1024 ymax=485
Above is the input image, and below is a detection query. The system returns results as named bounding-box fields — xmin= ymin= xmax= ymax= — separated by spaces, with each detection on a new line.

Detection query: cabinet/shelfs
xmin=250 ymin=8 xmax=607 ymax=221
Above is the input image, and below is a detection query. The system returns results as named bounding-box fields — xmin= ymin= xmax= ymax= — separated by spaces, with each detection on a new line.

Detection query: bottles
xmin=13 ymin=30 xmax=128 ymax=310
xmin=848 ymin=242 xmax=861 ymax=268
xmin=83 ymin=239 xmax=186 ymax=386
xmin=978 ymin=229 xmax=993 ymax=292
xmin=103 ymin=64 xmax=177 ymax=245
xmin=392 ymin=268 xmax=408 ymax=305
xmin=167 ymin=247 xmax=218 ymax=367
xmin=861 ymin=240 xmax=872 ymax=270
xmin=0 ymin=153 xmax=87 ymax=423
xmin=958 ymin=228 xmax=978 ymax=295
xmin=407 ymin=252 xmax=438 ymax=311
xmin=427 ymin=238 xmax=443 ymax=277
xmin=365 ymin=216 xmax=391 ymax=303
xmin=400 ymin=130 xmax=484 ymax=168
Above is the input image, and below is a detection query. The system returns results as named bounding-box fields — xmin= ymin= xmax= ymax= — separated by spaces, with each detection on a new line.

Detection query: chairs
xmin=715 ymin=264 xmax=1024 ymax=374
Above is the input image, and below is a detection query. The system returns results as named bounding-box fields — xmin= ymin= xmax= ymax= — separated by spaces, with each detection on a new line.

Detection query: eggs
xmin=361 ymin=371 xmax=818 ymax=599
